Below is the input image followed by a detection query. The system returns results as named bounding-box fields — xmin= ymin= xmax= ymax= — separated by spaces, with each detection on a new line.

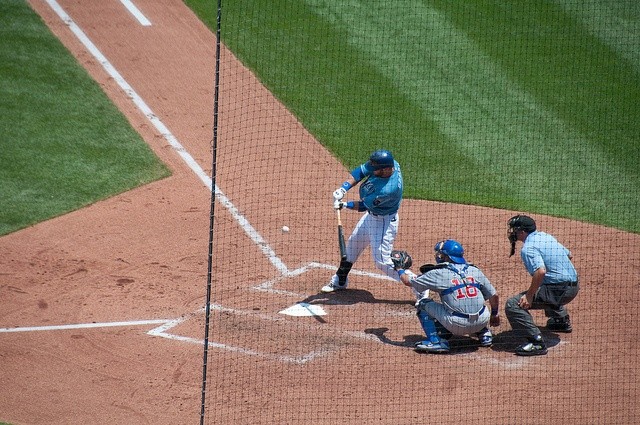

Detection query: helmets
xmin=365 ymin=150 xmax=394 ymax=171
xmin=507 ymin=215 xmax=536 ymax=244
xmin=434 ymin=240 xmax=467 ymax=264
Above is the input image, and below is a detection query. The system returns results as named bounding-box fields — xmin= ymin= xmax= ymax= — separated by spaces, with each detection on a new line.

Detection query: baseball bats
xmin=335 ymin=199 xmax=347 ymax=261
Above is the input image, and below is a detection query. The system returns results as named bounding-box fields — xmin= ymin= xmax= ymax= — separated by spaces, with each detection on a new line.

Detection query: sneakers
xmin=415 ymin=289 xmax=430 ymax=307
xmin=514 ymin=335 xmax=547 ymax=355
xmin=414 ymin=338 xmax=450 ymax=353
xmin=320 ymin=278 xmax=349 ymax=292
xmin=481 ymin=333 xmax=492 ymax=346
xmin=546 ymin=315 xmax=572 ymax=332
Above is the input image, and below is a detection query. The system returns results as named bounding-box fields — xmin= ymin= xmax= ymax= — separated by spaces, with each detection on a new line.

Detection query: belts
xmin=546 ymin=282 xmax=578 ymax=286
xmin=453 ymin=305 xmax=485 ymax=318
xmin=368 ymin=211 xmax=377 ymax=216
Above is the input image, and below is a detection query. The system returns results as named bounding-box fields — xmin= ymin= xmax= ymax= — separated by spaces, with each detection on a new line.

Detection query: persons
xmin=505 ymin=215 xmax=579 ymax=352
xmin=391 ymin=240 xmax=500 ymax=354
xmin=320 ymin=150 xmax=429 ymax=308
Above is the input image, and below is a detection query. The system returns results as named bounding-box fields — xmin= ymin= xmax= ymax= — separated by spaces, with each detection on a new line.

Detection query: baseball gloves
xmin=389 ymin=250 xmax=412 ymax=271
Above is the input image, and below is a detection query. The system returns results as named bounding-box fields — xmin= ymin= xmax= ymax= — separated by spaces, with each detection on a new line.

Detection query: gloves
xmin=334 ymin=200 xmax=347 ymax=209
xmin=333 ymin=187 xmax=347 ymax=201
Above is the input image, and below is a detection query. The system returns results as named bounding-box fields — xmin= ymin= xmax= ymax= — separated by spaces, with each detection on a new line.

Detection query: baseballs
xmin=282 ymin=226 xmax=289 ymax=234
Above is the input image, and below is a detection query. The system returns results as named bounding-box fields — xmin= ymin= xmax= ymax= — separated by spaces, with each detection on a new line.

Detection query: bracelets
xmin=491 ymin=307 xmax=499 ymax=315
